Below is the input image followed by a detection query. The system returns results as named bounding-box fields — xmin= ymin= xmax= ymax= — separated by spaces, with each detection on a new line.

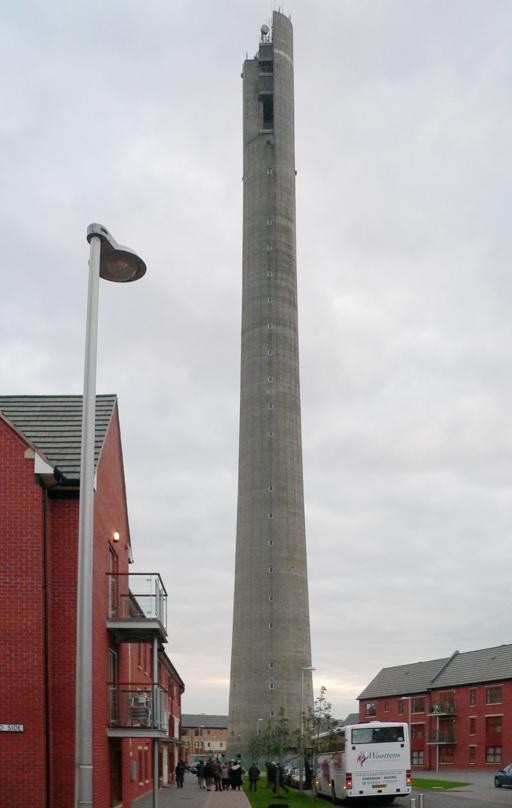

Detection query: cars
xmin=494 ymin=761 xmax=512 ymax=788
xmin=282 ymin=766 xmax=309 ymax=789
xmin=190 ymin=761 xmax=206 ymax=775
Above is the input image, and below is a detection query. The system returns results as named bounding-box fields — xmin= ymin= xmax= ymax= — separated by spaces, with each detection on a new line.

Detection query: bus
xmin=309 ymin=718 xmax=415 ymax=806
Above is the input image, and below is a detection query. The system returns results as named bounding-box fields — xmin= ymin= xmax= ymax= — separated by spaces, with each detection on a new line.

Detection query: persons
xmin=173 ymin=756 xmax=291 ymax=795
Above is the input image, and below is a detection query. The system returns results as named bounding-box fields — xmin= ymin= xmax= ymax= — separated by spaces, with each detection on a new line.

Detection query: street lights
xmin=400 ymin=695 xmax=412 ymax=735
xmin=69 ymin=221 xmax=150 ymax=807
xmin=298 ymin=666 xmax=320 ymax=794
xmin=197 ymin=724 xmax=205 ymax=761
xmin=256 ymin=717 xmax=264 ymax=735
